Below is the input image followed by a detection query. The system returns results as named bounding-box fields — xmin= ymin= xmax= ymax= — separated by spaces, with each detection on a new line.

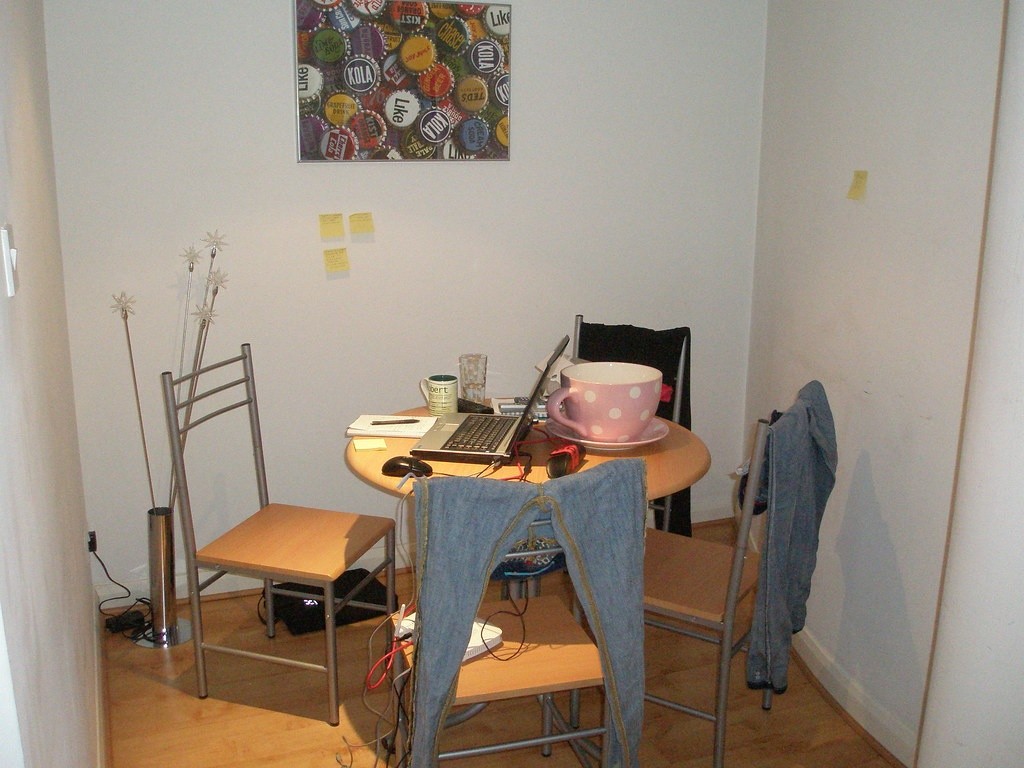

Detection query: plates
xmin=545 ymin=417 xmax=669 ymax=450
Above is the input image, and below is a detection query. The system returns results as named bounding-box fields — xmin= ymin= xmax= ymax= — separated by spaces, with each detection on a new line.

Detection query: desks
xmin=345 ymin=396 xmax=712 ymax=762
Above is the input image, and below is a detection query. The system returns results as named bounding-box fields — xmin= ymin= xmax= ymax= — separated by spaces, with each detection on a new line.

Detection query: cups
xmin=459 ymin=354 xmax=488 ymax=406
xmin=548 ymin=362 xmax=662 ymax=442
xmin=419 ymin=375 xmax=458 ymax=417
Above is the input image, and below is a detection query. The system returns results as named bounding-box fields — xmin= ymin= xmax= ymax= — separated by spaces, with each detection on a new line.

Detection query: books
xmin=346 ymin=415 xmax=438 ymax=439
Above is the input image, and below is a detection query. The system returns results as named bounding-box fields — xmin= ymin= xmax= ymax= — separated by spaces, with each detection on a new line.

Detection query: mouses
xmin=381 ymin=456 xmax=433 ymax=478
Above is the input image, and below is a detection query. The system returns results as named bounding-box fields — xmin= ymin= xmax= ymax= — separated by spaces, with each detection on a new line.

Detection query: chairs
xmin=573 ymin=315 xmax=688 ymax=529
xmin=568 ymin=379 xmax=825 ymax=768
xmin=161 ymin=344 xmax=397 ymax=726
xmin=389 ymin=459 xmax=647 ymax=768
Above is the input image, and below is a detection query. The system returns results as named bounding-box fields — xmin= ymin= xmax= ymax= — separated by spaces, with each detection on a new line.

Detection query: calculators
xmin=514 ymin=394 xmax=553 ymax=407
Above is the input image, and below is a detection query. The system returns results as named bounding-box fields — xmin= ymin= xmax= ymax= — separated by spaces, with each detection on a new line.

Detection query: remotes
xmin=457 ymin=398 xmax=494 ymax=415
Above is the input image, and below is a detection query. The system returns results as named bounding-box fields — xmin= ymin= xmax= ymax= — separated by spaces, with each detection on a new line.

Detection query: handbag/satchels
xmin=257 ymin=568 xmax=399 ymax=636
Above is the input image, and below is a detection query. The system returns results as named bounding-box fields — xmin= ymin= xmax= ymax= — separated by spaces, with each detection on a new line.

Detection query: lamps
xmin=109 ymin=228 xmax=230 ymax=648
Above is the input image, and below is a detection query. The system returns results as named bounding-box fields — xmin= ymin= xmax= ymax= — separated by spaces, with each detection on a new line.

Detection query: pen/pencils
xmin=370 ymin=419 xmax=421 ymax=425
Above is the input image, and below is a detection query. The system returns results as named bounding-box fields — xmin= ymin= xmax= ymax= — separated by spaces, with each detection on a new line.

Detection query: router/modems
xmin=393 ymin=605 xmax=502 ymax=663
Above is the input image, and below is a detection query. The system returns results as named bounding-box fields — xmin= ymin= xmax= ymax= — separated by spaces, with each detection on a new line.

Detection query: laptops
xmin=410 ymin=334 xmax=570 ymax=465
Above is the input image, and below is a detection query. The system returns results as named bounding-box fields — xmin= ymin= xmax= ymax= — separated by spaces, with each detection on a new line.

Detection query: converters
xmin=106 ymin=610 xmax=144 ymax=633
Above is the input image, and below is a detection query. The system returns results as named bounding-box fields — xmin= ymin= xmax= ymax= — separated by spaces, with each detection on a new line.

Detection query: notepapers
xmin=351 ymin=439 xmax=387 ymax=451
